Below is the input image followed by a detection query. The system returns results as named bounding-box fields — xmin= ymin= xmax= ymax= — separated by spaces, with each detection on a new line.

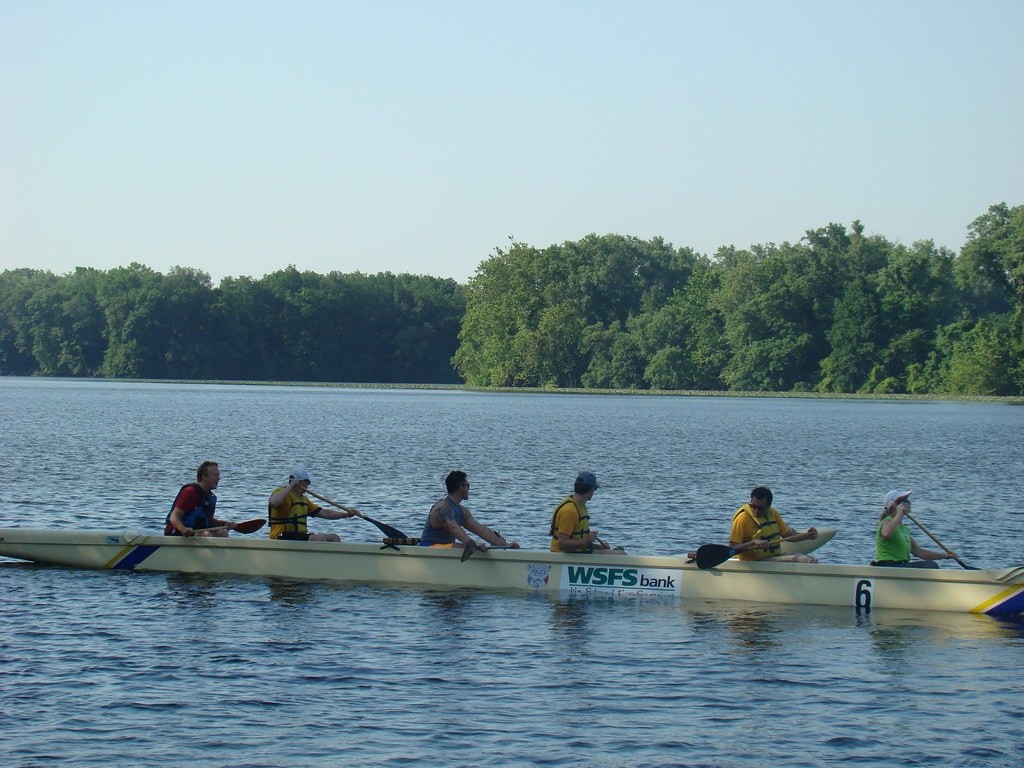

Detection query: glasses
xmin=463 ymin=483 xmax=470 ymax=489
xmin=594 ymin=487 xmax=597 ymax=491
xmin=750 ymin=502 xmax=768 ymax=511
xmin=898 ymin=498 xmax=908 ymax=504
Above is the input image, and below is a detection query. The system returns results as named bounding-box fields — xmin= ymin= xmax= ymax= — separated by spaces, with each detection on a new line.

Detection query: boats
xmin=0 ymin=525 xmax=1023 ymax=621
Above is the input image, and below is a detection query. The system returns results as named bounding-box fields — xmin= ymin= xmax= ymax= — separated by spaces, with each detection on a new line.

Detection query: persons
xmin=551 ymin=471 xmax=628 ymax=555
xmin=164 ymin=460 xmax=237 ymax=537
xmin=728 ymin=486 xmax=818 ymax=563
xmin=874 ymin=490 xmax=960 ymax=569
xmin=419 ymin=471 xmax=520 ymax=552
xmin=268 ymin=468 xmax=362 ymax=542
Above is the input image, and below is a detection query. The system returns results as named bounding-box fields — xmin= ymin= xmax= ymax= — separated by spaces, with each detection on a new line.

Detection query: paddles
xmin=693 ymin=528 xmax=819 ymax=571
xmin=899 ymin=507 xmax=982 ymax=571
xmin=456 ymin=542 xmax=519 ymax=561
xmin=591 ymin=532 xmax=620 ymax=555
xmin=176 ymin=516 xmax=269 ymax=536
xmin=298 ymin=485 xmax=407 ymax=540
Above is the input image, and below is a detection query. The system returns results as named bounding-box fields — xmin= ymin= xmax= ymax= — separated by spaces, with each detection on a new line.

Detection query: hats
xmin=289 ymin=469 xmax=316 ymax=485
xmin=883 ymin=490 xmax=912 ymax=512
xmin=576 ymin=472 xmax=601 ymax=488
